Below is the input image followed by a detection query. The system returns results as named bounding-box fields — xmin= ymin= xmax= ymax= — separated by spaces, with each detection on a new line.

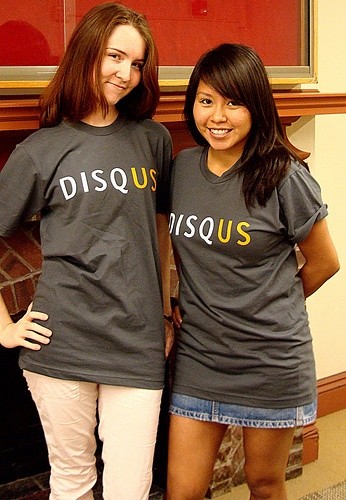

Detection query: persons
xmin=166 ymin=44 xmax=341 ymax=500
xmin=1 ymin=1 xmax=174 ymax=500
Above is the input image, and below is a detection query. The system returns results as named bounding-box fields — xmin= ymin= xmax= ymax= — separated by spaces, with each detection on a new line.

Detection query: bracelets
xmin=163 ymin=315 xmax=173 ymax=324
